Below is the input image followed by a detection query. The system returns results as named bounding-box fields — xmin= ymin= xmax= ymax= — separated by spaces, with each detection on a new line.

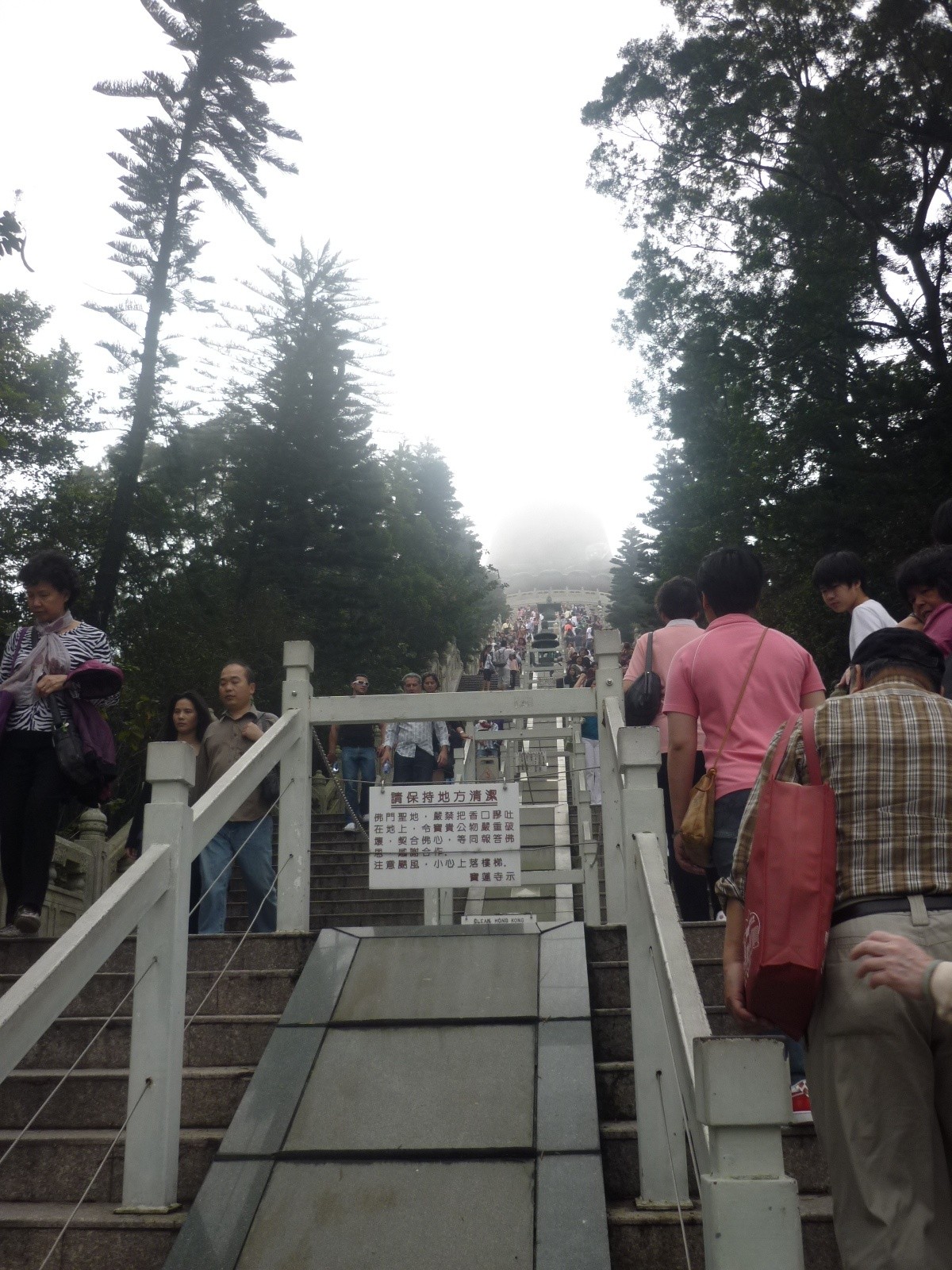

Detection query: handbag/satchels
xmin=52 ymin=719 xmax=117 ymax=786
xmin=259 ymin=770 xmax=281 ymax=801
xmin=624 ymin=671 xmax=662 ymax=726
xmin=682 ymin=767 xmax=717 ymax=867
xmin=742 ymin=707 xmax=838 ymax=1042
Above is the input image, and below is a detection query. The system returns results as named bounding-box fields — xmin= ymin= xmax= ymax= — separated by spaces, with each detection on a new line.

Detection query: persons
xmin=0 ymin=552 xmax=121 ymax=934
xmin=126 ymin=550 xmax=952 ymax=1122
xmin=711 ymin=625 xmax=952 ymax=1270
xmin=847 ymin=931 xmax=952 ymax=1019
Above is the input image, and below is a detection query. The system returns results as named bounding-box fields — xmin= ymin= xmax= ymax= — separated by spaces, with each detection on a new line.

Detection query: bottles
xmin=383 ymin=760 xmax=391 ymax=774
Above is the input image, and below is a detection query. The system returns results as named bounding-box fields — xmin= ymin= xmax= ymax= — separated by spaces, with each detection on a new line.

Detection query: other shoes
xmin=344 ymin=822 xmax=356 ymax=833
xmin=13 ymin=902 xmax=41 ymax=931
xmin=0 ymin=920 xmax=39 ymax=939
xmin=786 ymin=1077 xmax=813 ymax=1126
xmin=357 ymin=813 xmax=369 ymax=823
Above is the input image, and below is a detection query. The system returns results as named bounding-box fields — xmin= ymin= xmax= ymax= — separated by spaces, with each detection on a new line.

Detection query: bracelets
xmin=917 ymin=958 xmax=942 ymax=1012
xmin=459 ymin=731 xmax=464 ymax=734
xmin=672 ymin=830 xmax=681 ymax=838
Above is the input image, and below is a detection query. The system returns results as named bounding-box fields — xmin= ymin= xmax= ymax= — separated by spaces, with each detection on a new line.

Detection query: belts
xmin=830 ymin=891 xmax=952 ymax=927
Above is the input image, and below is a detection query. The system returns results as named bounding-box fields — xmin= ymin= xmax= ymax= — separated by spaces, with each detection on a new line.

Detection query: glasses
xmin=352 ymin=680 xmax=369 ymax=687
